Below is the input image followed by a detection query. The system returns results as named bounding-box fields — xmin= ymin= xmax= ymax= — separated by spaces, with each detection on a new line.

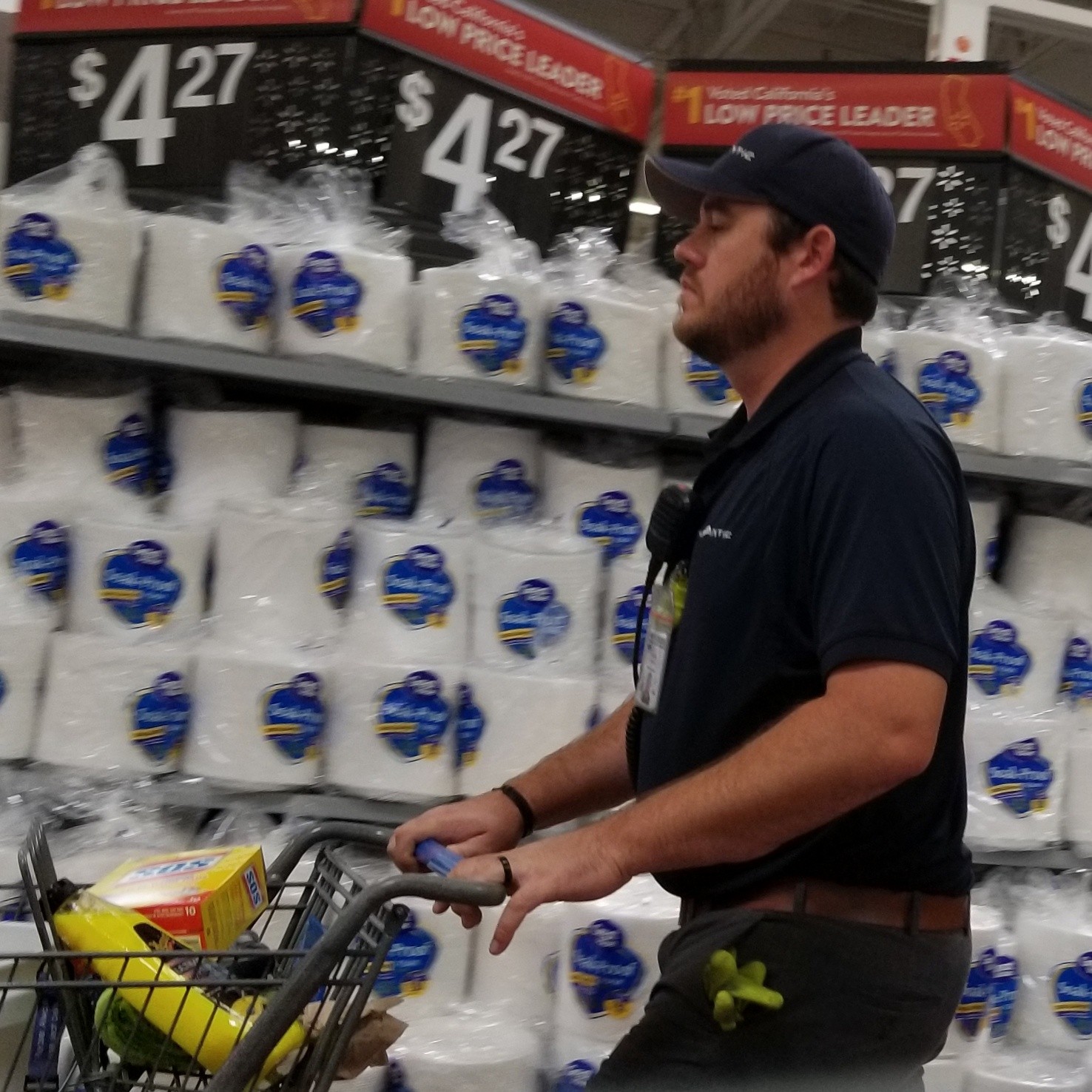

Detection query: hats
xmin=642 ymin=123 xmax=895 ymax=277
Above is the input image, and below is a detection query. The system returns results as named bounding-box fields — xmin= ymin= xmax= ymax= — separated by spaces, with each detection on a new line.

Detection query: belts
xmin=678 ymin=878 xmax=970 ymax=925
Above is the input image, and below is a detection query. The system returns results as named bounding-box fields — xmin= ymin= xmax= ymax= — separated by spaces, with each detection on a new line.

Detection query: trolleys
xmin=0 ymin=815 xmax=508 ymax=1091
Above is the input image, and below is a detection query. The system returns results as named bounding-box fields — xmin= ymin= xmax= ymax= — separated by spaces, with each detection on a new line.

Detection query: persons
xmin=389 ymin=126 xmax=974 ymax=1092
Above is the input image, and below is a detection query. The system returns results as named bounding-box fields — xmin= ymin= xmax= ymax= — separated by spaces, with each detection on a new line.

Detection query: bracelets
xmin=491 ymin=785 xmax=534 ymax=838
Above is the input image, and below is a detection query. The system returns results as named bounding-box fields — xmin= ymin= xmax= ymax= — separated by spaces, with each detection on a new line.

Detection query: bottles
xmin=38 ymin=878 xmax=309 ymax=1092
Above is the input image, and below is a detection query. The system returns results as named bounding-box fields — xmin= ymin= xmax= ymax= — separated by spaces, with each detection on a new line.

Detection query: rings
xmin=499 ymin=857 xmax=513 ymax=885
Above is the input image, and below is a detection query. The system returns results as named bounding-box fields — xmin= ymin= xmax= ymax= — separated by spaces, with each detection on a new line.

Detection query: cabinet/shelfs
xmin=1 ymin=308 xmax=1091 ymax=822
